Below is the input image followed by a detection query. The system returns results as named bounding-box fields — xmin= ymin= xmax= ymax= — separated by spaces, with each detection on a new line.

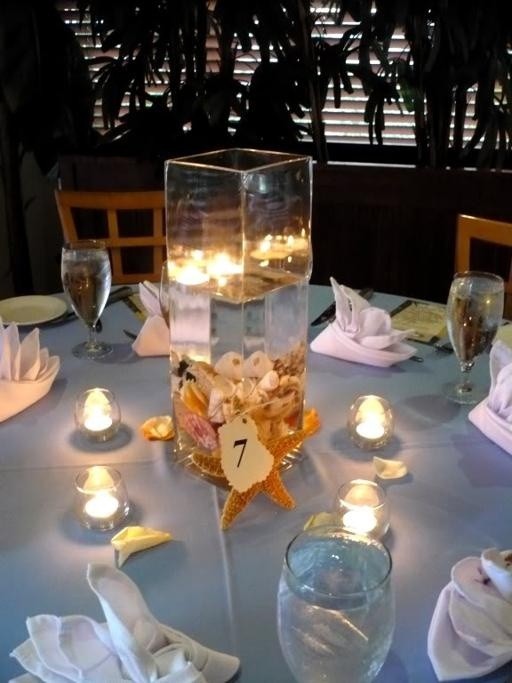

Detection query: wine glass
xmin=277 ymin=527 xmax=392 ymax=682
xmin=440 ymin=271 xmax=503 ymax=403
xmin=62 ymin=240 xmax=113 ymax=360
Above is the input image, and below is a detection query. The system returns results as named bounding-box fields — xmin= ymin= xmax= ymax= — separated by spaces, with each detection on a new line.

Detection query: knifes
xmin=65 ymin=285 xmax=133 ymax=323
xmin=310 ymin=286 xmax=373 ymax=327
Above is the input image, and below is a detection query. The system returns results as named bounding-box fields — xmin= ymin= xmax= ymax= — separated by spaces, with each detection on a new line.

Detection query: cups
xmin=335 ymin=479 xmax=391 ymax=541
xmin=75 ymin=467 xmax=130 ymax=532
xmin=75 ymin=388 xmax=120 ymax=442
xmin=347 ymin=395 xmax=392 ymax=448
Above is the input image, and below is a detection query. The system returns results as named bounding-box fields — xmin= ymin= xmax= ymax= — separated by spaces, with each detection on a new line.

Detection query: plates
xmin=0 ymin=295 xmax=66 ymax=328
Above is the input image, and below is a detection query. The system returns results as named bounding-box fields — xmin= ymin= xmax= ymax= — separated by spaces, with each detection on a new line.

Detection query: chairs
xmin=55 ymin=186 xmax=169 ymax=286
xmin=455 ymin=208 xmax=511 ymax=324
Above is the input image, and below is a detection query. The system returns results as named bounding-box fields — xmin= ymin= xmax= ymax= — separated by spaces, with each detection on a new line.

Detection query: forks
xmin=433 ymin=340 xmax=453 ymax=354
